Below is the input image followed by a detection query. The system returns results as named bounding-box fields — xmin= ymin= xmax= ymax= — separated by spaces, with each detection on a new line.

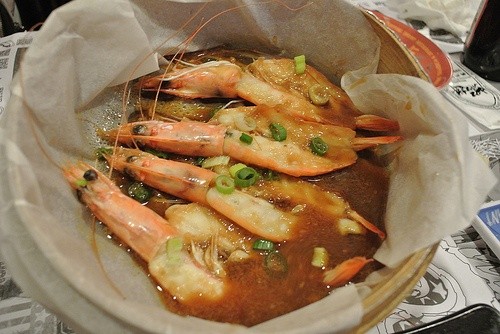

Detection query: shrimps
xmin=60 ymin=0 xmax=406 ymax=310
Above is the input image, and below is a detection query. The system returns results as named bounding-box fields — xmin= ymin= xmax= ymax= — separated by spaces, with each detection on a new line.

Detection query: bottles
xmin=460 ymin=0 xmax=500 ymax=83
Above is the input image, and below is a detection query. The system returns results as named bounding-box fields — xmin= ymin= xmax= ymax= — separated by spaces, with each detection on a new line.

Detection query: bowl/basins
xmin=0 ymin=0 xmax=442 ymax=334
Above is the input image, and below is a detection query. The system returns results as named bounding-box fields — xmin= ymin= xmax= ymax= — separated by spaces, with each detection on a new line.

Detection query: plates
xmin=366 ymin=9 xmax=453 ymax=91
